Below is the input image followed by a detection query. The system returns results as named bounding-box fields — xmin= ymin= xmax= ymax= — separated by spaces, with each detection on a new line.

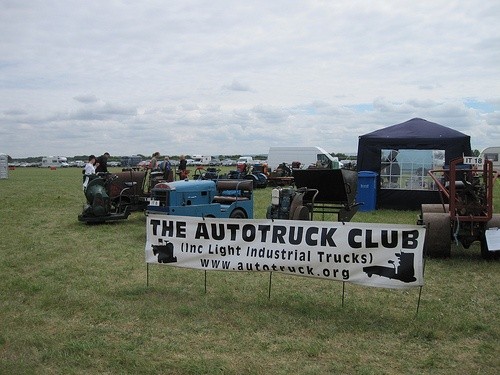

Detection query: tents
xmin=358 ymin=118 xmax=471 ymax=211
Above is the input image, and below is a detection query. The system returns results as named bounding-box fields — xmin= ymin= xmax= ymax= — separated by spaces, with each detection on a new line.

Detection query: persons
xmin=159 ymin=156 xmax=170 ymax=171
xmin=83 ymin=155 xmax=99 ymax=187
xmin=149 ymin=152 xmax=159 ymax=169
xmin=178 ymin=155 xmax=187 ymax=180
xmin=95 ymin=153 xmax=109 ymax=173
xmin=386 ymin=149 xmax=399 ymax=183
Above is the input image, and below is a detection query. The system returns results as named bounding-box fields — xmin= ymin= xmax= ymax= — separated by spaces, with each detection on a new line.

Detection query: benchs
xmin=214 ymin=180 xmax=253 ymax=204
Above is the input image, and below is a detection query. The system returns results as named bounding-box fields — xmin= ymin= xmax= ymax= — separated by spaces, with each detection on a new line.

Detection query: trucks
xmin=41 ymin=156 xmax=69 ymax=168
xmin=269 ymin=146 xmax=343 ymax=173
xmin=238 ymin=157 xmax=252 ymax=166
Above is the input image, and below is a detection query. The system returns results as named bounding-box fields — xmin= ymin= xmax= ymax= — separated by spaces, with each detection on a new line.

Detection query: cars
xmin=6 ymin=160 xmax=270 ymax=167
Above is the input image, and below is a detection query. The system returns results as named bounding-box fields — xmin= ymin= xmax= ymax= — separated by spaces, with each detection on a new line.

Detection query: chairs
xmin=150 ymin=162 xmax=167 ymax=176
xmin=205 ymin=168 xmax=217 ymax=177
